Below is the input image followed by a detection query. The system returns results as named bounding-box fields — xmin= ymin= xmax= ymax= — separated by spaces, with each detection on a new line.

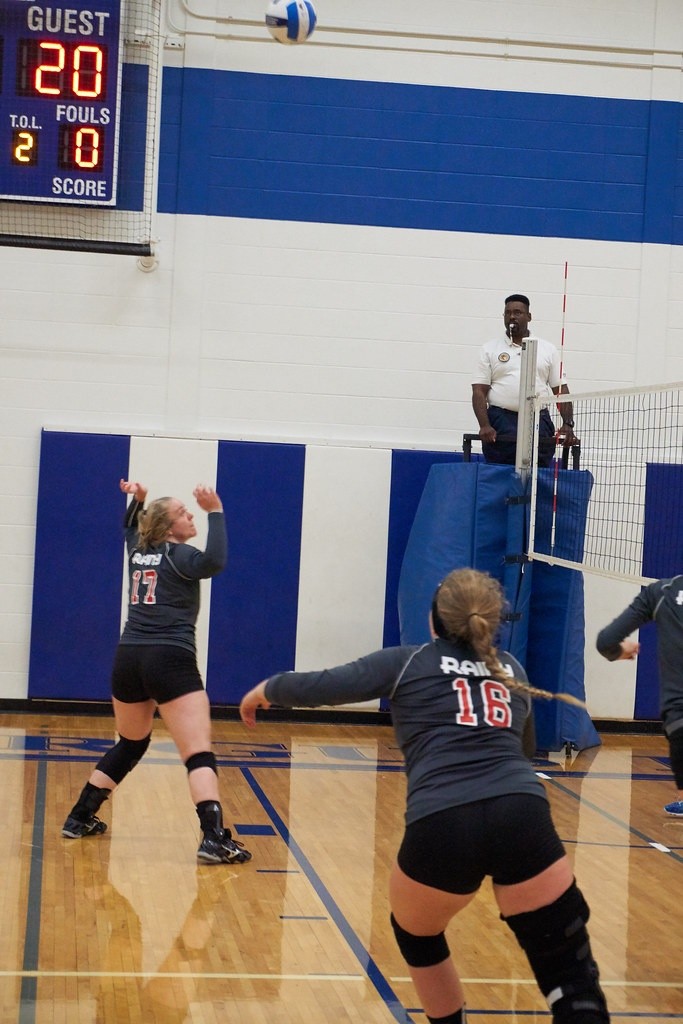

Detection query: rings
xmin=572 ymin=437 xmax=575 ymax=439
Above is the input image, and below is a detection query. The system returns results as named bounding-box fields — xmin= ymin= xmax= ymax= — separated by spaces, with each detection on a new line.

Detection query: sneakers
xmin=62 ymin=806 xmax=107 ymax=838
xmin=197 ymin=828 xmax=253 ymax=863
xmin=664 ymin=799 xmax=683 ymax=815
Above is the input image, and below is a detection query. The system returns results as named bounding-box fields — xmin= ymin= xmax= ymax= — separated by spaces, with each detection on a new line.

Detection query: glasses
xmin=503 ymin=311 xmax=527 ymax=317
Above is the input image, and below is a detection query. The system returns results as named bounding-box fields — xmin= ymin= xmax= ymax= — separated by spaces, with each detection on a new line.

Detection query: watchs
xmin=564 ymin=419 xmax=574 ymax=427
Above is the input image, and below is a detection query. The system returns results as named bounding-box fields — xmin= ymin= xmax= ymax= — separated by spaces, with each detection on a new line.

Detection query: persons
xmin=598 ymin=575 xmax=683 ymax=817
xmin=58 ymin=837 xmax=231 ymax=1024
xmin=240 ymin=567 xmax=610 ymax=1024
xmin=471 ymin=295 xmax=577 ymax=467
xmin=60 ymin=479 xmax=253 ymax=864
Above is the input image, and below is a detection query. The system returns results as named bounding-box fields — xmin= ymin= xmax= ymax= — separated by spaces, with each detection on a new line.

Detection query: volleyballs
xmin=264 ymin=0 xmax=317 ymax=45
